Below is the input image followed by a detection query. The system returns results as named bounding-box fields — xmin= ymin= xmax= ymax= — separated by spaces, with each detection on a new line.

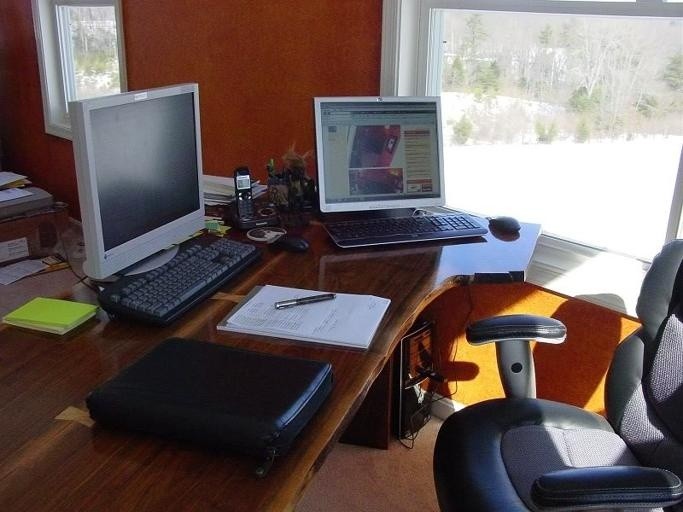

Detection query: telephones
xmin=231 ymin=166 xmax=281 ymax=230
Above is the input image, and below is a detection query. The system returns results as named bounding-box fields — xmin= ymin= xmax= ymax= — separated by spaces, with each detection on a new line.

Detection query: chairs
xmin=432 ymin=239 xmax=683 ymax=512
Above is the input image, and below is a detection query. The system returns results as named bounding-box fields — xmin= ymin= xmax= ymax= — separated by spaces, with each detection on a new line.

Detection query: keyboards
xmin=320 ymin=212 xmax=488 ymax=249
xmin=97 ymin=234 xmax=264 ymax=326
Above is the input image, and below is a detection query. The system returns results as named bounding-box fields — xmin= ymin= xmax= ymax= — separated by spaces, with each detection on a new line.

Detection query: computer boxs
xmin=391 ymin=317 xmax=436 ymax=440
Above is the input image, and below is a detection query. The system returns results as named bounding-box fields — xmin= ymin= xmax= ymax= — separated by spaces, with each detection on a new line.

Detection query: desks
xmin=0 ymin=197 xmax=542 ymax=512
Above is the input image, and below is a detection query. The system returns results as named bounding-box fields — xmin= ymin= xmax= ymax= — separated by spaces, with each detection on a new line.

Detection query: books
xmin=215 ymin=282 xmax=390 ymax=350
xmin=203 ymin=174 xmax=269 ymax=206
xmin=1 ymin=171 xmax=53 ymax=219
xmin=1 ymin=252 xmax=69 ymax=286
xmin=2 ymin=296 xmax=100 ymax=338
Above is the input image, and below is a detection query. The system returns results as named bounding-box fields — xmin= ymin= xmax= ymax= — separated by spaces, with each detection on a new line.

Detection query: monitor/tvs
xmin=313 ymin=96 xmax=446 ymax=217
xmin=67 ymin=82 xmax=207 ymax=280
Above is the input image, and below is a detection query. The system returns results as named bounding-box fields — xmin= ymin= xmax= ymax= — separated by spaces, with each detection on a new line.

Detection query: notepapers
xmin=2 ymin=296 xmax=100 ymax=336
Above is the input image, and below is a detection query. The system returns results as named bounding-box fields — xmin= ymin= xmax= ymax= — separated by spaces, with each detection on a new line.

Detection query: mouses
xmin=272 ymin=235 xmax=311 ymax=252
xmin=489 ymin=216 xmax=521 ymax=233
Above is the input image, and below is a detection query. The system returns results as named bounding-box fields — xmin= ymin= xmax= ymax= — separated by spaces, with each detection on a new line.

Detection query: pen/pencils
xmin=274 ymin=293 xmax=336 ymax=310
